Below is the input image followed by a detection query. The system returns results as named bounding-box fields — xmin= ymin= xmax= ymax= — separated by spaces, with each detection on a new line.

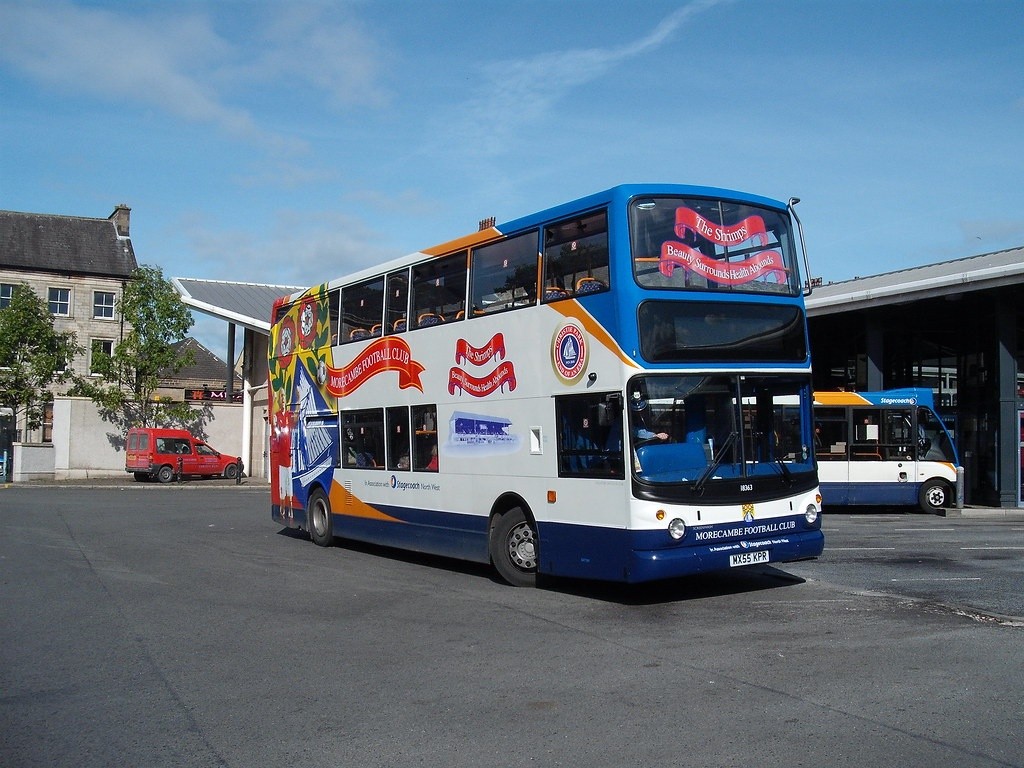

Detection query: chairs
xmin=332 ymin=278 xmax=611 ymax=345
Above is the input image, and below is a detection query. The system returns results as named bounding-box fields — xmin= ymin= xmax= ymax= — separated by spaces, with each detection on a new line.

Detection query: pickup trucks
xmin=125 ymin=427 xmax=243 ymax=483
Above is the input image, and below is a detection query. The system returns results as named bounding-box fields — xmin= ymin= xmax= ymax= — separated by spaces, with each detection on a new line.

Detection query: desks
xmin=416 ymin=430 xmax=436 ymax=436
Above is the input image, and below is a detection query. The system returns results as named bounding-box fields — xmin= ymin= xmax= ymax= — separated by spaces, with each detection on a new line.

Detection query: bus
xmin=0 ymin=406 xmax=16 ymax=477
xmin=645 ymin=388 xmax=960 ymax=514
xmin=266 ymin=182 xmax=824 ymax=590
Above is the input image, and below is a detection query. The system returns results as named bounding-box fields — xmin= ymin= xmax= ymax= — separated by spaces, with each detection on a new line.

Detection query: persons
xmin=355 ymin=443 xmax=377 ymax=467
xmin=606 ymin=409 xmax=669 ymax=463
xmin=426 ymin=444 xmax=438 ymax=471
xmin=396 ymin=456 xmax=410 ymax=469
